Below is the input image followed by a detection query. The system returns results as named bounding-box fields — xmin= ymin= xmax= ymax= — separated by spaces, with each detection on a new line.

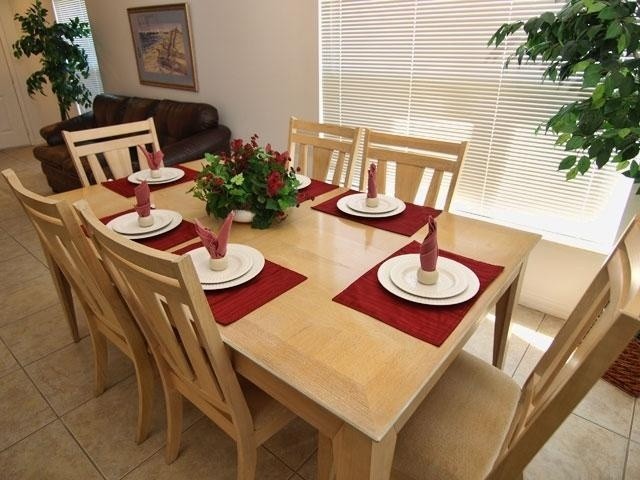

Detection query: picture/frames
xmin=125 ymin=2 xmax=200 ymax=93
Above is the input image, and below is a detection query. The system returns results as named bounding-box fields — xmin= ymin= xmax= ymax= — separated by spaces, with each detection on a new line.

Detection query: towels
xmin=416 ymin=212 xmax=445 ymax=273
xmin=188 ymin=208 xmax=238 ymax=259
xmin=135 ymin=142 xmax=165 ymax=170
xmin=365 ymin=161 xmax=377 ymax=199
xmin=133 ymin=179 xmax=152 ymax=218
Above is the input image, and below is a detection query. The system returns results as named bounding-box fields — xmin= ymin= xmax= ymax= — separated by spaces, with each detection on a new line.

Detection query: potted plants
xmin=486 ymin=0 xmax=640 ymax=404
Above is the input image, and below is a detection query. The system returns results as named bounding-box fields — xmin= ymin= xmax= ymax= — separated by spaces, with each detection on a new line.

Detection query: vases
xmin=229 ymin=208 xmax=259 ymax=228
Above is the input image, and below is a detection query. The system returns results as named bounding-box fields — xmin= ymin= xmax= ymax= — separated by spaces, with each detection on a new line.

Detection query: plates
xmin=128 ymin=168 xmax=185 ymax=184
xmin=288 ymin=174 xmax=311 ymax=189
xmin=390 ymin=260 xmax=469 ymax=298
xmin=337 ymin=193 xmax=406 ymax=218
xmin=106 ymin=210 xmax=182 ymax=239
xmin=295 ymin=176 xmax=303 ymax=186
xmin=113 ymin=212 xmax=173 ymax=234
xmin=192 ymin=248 xmax=254 ymax=283
xmin=347 ymin=198 xmax=398 ymax=213
xmin=136 ymin=169 xmax=181 ymax=182
xmin=184 ymin=243 xmax=265 ymax=290
xmin=377 ymin=254 xmax=480 ymax=306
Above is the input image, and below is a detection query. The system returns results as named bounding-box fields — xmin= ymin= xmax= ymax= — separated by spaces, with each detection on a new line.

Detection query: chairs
xmin=61 ymin=116 xmax=168 ymax=192
xmin=0 ymin=166 xmax=159 ymax=449
xmin=69 ymin=196 xmax=303 ymax=480
xmin=280 ymin=114 xmax=362 ymax=201
xmin=359 ymin=124 xmax=470 ymax=219
xmin=391 ymin=211 xmax=639 ymax=480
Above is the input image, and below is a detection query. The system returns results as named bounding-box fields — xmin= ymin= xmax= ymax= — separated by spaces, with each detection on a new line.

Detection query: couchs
xmin=31 ymin=90 xmax=234 ymax=197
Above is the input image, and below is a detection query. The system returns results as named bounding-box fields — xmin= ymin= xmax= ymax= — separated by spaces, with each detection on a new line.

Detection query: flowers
xmin=183 ymin=131 xmax=319 ymax=231
